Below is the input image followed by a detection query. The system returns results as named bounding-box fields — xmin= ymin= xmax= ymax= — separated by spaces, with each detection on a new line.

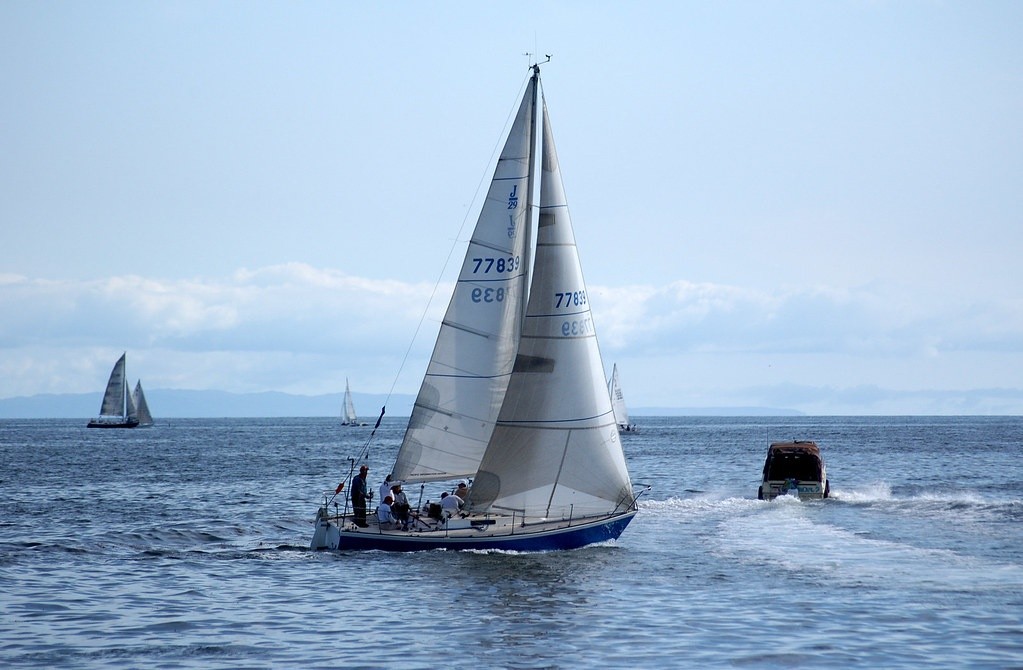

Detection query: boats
xmin=758 ymin=440 xmax=830 ymax=498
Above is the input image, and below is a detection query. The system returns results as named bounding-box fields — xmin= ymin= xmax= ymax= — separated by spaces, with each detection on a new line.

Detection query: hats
xmin=360 ymin=466 xmax=369 ymax=471
xmin=389 ymin=481 xmax=400 ymax=490
xmin=385 ymin=496 xmax=396 ymax=502
xmin=458 ymin=479 xmax=467 ymax=487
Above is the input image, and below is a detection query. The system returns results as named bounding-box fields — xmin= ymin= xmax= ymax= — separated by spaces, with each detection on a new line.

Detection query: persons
xmin=454 ymin=483 xmax=469 ymax=509
xmin=377 ymin=495 xmax=402 ymax=525
xmin=390 ymin=485 xmax=408 ymax=513
xmin=439 ymin=492 xmax=465 ymax=523
xmin=351 ymin=465 xmax=369 ymax=527
xmin=380 ymin=474 xmax=393 ymax=503
xmin=632 ymin=425 xmax=637 ymax=431
xmin=620 ymin=425 xmax=625 ymax=430
xmin=626 ymin=425 xmax=630 ymax=431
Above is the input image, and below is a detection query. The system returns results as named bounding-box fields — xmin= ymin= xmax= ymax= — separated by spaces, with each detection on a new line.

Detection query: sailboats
xmin=340 ymin=377 xmax=369 ymax=427
xmin=310 ymin=52 xmax=652 ymax=553
xmin=607 ymin=363 xmax=638 ymax=435
xmin=86 ymin=351 xmax=155 ymax=428
